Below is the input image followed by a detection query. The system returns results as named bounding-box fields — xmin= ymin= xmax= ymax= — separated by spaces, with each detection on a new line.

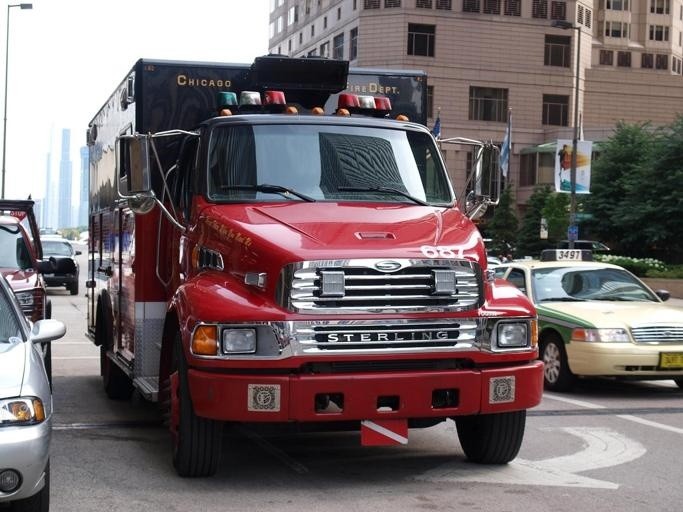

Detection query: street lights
xmin=1 ymin=2 xmax=33 ymax=200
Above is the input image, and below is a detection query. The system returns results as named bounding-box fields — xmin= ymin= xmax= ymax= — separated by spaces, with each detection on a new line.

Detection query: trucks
xmin=84 ymin=53 xmax=545 ymax=478
xmin=0 ymin=199 xmax=52 ymax=393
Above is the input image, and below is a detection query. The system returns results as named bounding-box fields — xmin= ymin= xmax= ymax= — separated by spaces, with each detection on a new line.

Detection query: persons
xmin=498 ymin=254 xmax=514 ymax=263
xmin=558 ymin=145 xmax=569 ymax=176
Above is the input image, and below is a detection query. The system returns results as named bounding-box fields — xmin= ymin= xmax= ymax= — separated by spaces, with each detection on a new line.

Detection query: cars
xmin=482 ymin=239 xmax=683 ymax=392
xmin=0 ymin=274 xmax=66 ymax=511
xmin=40 ymin=235 xmax=81 ymax=295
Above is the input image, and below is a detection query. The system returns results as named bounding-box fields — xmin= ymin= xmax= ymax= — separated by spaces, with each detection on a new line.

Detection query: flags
xmin=500 ymin=120 xmax=511 ymax=177
xmin=431 ymin=114 xmax=442 ymax=141
